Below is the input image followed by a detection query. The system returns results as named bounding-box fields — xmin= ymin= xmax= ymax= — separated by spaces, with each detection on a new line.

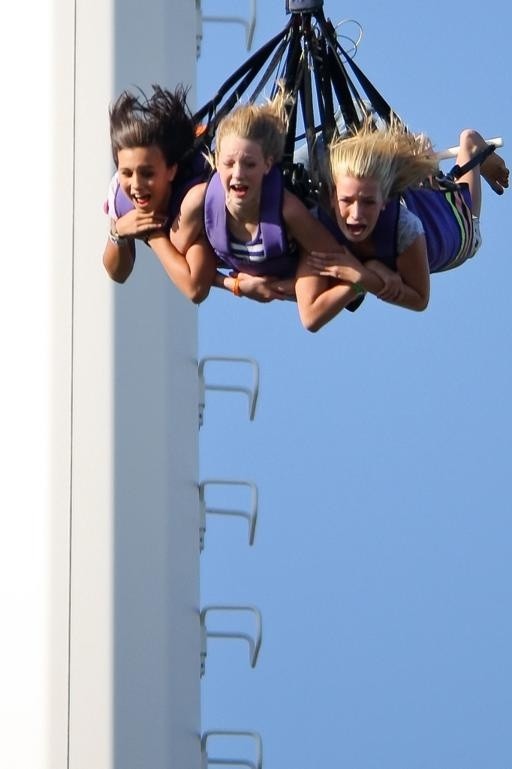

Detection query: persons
xmin=293 ymin=105 xmax=512 ymax=335
xmin=100 ymin=79 xmax=217 ymax=307
xmin=166 ymin=79 xmax=378 ymax=306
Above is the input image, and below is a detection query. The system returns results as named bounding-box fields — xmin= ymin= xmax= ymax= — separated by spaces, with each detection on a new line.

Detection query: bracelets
xmin=231 ymin=277 xmax=245 ymax=297
xmin=350 ymin=280 xmax=364 ymax=297
xmin=107 ymin=220 xmax=130 ymax=250
